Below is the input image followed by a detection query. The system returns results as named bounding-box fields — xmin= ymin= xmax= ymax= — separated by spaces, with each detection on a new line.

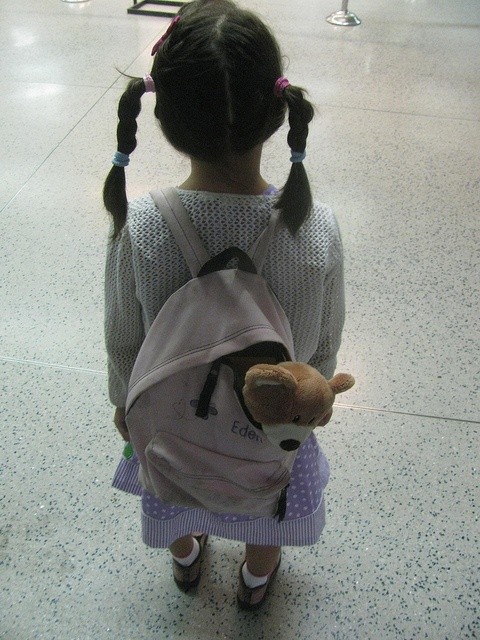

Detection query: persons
xmin=103 ymin=1 xmax=345 ymax=609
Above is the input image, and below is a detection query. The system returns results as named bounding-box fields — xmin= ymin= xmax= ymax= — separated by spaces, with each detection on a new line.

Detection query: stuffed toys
xmin=239 ymin=361 xmax=354 ymax=452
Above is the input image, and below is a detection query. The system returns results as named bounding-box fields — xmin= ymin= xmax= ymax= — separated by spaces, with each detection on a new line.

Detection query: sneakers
xmin=172 ymin=532 xmax=208 ymax=589
xmin=237 ymin=552 xmax=281 ymax=611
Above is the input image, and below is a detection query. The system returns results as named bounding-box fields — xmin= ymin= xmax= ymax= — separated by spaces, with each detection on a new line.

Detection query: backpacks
xmin=124 ymin=186 xmax=355 ymax=522
